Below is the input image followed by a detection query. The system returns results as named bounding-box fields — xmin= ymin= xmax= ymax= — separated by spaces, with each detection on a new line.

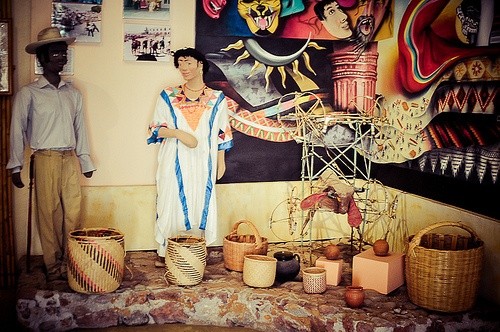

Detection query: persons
xmin=148 ymin=0 xmax=158 ymax=13
xmin=130 ymin=37 xmax=172 ymax=61
xmin=87 ymin=20 xmax=99 ymax=36
xmin=6 ymin=29 xmax=95 ymax=286
xmin=145 ymin=49 xmax=233 ymax=267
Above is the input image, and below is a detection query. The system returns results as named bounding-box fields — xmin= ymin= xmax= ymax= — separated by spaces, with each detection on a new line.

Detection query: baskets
xmin=222 ymin=221 xmax=267 ymax=272
xmin=67 ymin=227 xmax=124 ymax=294
xmin=165 ymin=236 xmax=207 ymax=286
xmin=404 ymin=222 xmax=484 ymax=313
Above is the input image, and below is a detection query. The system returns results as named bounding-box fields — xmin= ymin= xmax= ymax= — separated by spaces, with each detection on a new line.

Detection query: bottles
xmin=345 ymin=286 xmax=365 ymax=308
xmin=273 ymin=251 xmax=301 ymax=284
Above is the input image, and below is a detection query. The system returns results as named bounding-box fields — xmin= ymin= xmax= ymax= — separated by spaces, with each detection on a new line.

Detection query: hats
xmin=25 ymin=27 xmax=76 ymax=54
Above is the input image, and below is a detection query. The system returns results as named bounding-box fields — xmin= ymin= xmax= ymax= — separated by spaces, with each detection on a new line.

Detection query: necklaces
xmin=183 ymin=82 xmax=207 ymax=114
